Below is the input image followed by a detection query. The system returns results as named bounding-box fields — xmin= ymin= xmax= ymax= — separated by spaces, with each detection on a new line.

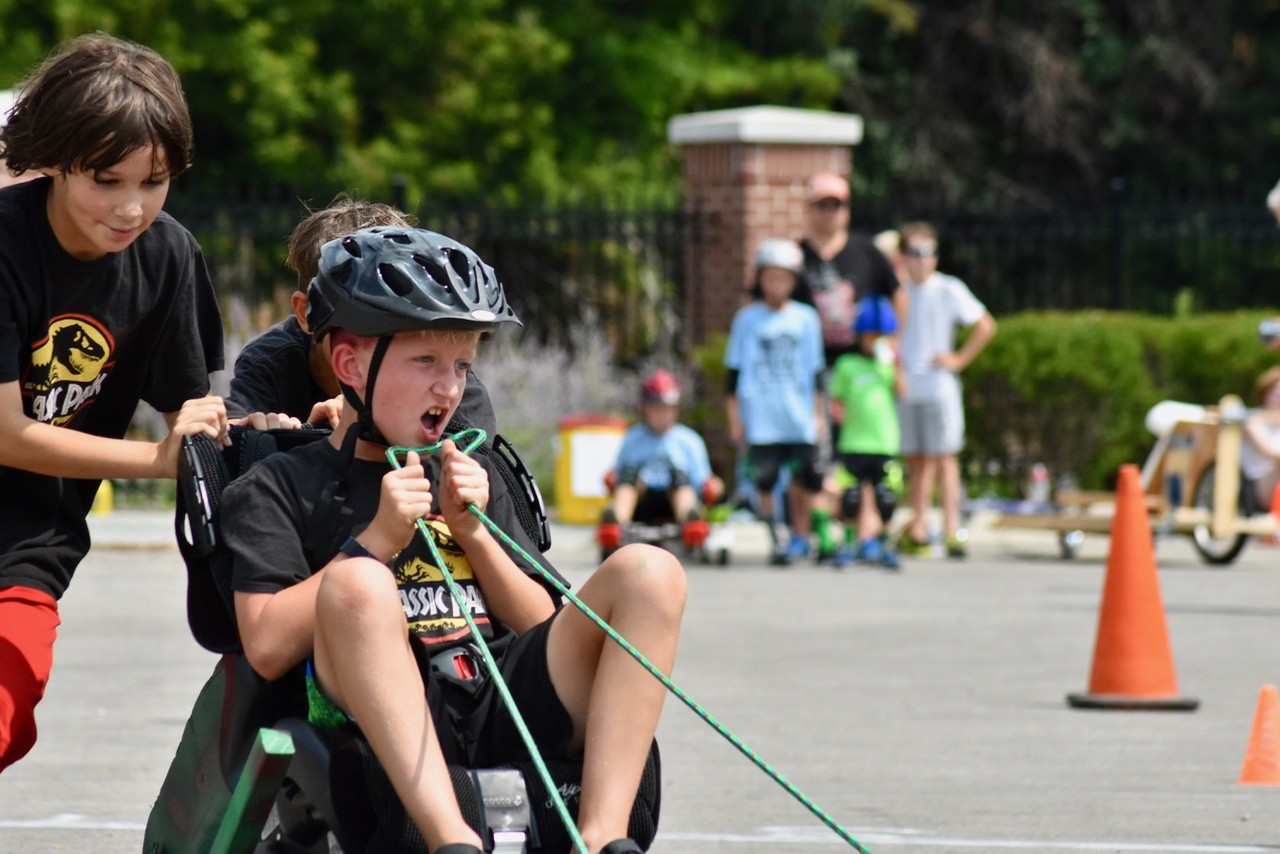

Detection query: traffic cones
xmin=1064 ymin=464 xmax=1203 ymax=713
xmin=1238 ymin=677 xmax=1280 ymax=788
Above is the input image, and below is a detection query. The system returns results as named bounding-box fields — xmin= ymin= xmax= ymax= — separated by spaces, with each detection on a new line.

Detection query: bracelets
xmin=337 ymin=535 xmax=376 ymax=560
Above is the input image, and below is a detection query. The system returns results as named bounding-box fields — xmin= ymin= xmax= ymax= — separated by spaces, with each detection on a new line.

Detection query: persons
xmin=598 ymin=368 xmax=722 ymax=545
xmin=215 ymin=190 xmax=688 ymax=854
xmin=1243 ymin=367 xmax=1280 ymax=512
xmin=0 ymin=28 xmax=303 ymax=768
xmin=723 ymin=173 xmax=996 ymax=573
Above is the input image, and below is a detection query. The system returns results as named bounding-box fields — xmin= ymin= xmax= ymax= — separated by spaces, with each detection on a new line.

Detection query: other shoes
xmin=775 ymin=535 xmax=966 ymax=568
xmin=684 ymin=518 xmax=709 ymax=549
xmin=597 ymin=521 xmax=619 ymax=549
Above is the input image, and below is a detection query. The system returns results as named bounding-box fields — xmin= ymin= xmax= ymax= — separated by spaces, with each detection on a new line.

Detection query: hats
xmin=806 ymin=173 xmax=849 ymax=204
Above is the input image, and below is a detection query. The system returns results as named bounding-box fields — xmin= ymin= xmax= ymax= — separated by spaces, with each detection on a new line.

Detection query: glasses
xmin=904 ymin=247 xmax=938 ymax=259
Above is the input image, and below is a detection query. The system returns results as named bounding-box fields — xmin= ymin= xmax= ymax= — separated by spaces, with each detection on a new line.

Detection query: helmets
xmin=306 ymin=226 xmax=523 ymax=342
xmin=849 ymin=299 xmax=892 ymax=332
xmin=752 ymin=238 xmax=806 ymax=276
xmin=639 ymin=371 xmax=682 ymax=407
